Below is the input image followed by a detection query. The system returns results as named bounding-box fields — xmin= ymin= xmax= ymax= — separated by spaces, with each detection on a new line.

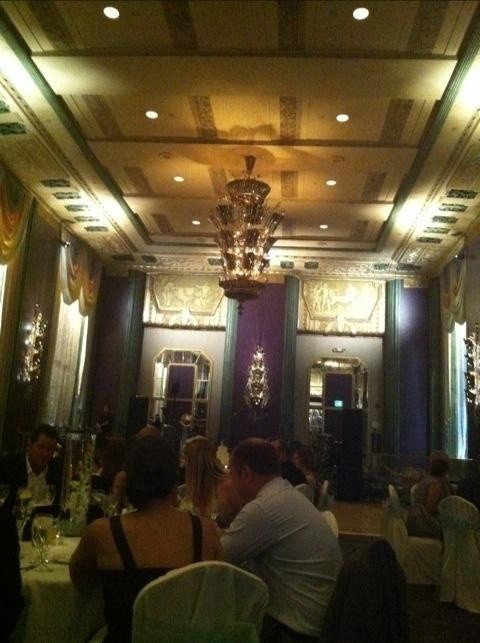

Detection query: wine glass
xmin=0 ymin=481 xmax=134 ymax=572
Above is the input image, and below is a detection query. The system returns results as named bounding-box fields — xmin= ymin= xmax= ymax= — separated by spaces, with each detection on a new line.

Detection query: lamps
xmin=208 ymin=155 xmax=281 ymax=301
xmin=246 ymin=344 xmax=269 ymax=409
xmin=464 ymin=331 xmax=480 ymax=407
xmin=16 ymin=311 xmax=48 ymax=382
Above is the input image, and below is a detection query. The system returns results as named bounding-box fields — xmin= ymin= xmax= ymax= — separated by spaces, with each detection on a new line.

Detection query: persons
xmin=457 ymin=454 xmax=479 ymax=509
xmin=0 ymin=406 xmax=347 ymax=643
xmin=406 ymin=451 xmax=453 ymax=538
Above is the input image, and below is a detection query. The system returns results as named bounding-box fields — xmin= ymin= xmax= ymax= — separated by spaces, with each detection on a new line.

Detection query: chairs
xmin=293 ymin=479 xmax=336 ymax=533
xmin=438 ymin=496 xmax=480 ymax=615
xmin=388 ymin=484 xmax=443 ymax=586
xmin=131 ymin=560 xmax=268 ymax=643
xmin=319 ymin=542 xmax=408 ymax=643
